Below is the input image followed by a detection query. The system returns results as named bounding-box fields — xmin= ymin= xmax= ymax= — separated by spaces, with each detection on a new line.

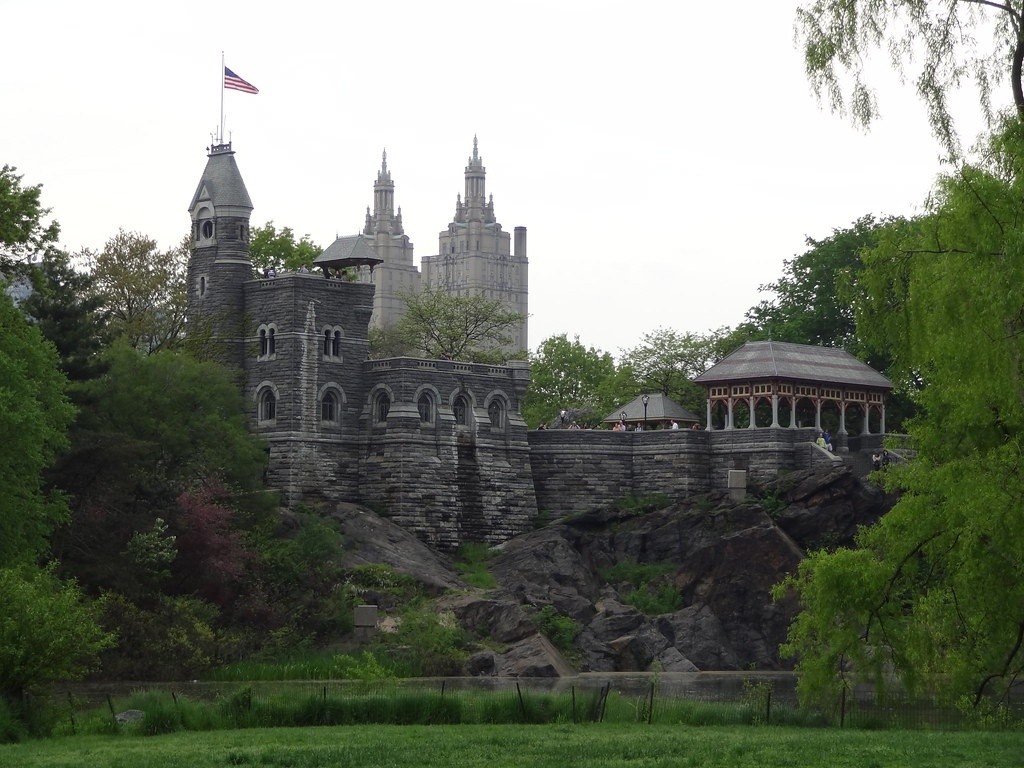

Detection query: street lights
xmin=641 ymin=393 xmax=650 ymax=430
xmin=618 ymin=410 xmax=628 ymax=426
xmin=560 ymin=410 xmax=567 ymax=429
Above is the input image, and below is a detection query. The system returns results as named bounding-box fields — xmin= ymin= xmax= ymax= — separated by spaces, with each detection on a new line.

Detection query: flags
xmin=223 ymin=66 xmax=259 ymax=96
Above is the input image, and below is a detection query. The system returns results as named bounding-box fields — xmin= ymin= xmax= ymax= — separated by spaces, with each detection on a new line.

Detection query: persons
xmin=299 ymin=263 xmax=307 ymax=272
xmin=816 ymin=432 xmax=828 ymax=450
xmin=568 ymin=421 xmax=580 ymax=430
xmin=634 ymin=423 xmax=643 ymax=431
xmin=613 ymin=423 xmax=620 ymax=431
xmin=264 ymin=267 xmax=277 ymax=278
xmin=538 ymin=422 xmax=549 ymax=430
xmin=618 ymin=420 xmax=626 ymax=431
xmin=335 ymin=271 xmax=341 ymax=279
xmin=671 ymin=420 xmax=678 ymax=429
xmin=822 ymin=429 xmax=833 ymax=451
xmin=872 ymin=453 xmax=881 ymax=471
xmin=882 ymin=450 xmax=892 ymax=472
